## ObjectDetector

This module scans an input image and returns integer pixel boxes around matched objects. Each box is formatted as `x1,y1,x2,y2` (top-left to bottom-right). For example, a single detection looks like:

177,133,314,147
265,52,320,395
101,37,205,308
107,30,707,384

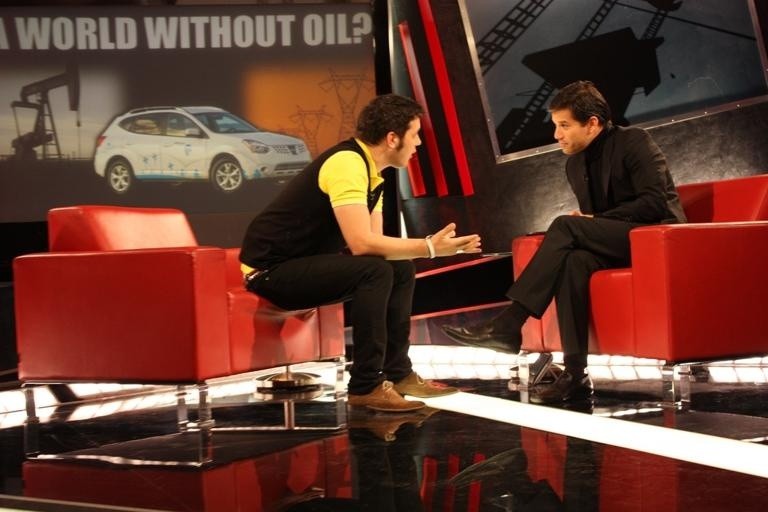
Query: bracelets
426,234,435,260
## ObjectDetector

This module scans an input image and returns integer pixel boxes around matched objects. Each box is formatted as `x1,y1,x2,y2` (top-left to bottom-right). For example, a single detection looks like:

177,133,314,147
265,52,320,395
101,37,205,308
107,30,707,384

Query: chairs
13,205,346,471
512,173,768,412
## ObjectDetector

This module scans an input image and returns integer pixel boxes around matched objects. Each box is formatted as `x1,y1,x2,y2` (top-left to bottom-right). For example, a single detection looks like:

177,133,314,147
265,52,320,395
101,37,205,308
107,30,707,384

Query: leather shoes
348,370,459,412
441,319,522,354
530,370,594,403
348,407,441,441
448,448,527,488
540,399,593,415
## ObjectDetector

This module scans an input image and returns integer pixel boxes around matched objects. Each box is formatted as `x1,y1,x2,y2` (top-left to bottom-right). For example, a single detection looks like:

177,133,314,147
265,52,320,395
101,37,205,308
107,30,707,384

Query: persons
343,403,440,510
240,93,481,412
440,82,688,406
448,404,597,511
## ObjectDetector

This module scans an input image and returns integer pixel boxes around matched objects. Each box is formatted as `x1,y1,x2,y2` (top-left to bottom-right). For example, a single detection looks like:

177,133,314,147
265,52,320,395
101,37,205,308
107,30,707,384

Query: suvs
91,103,314,192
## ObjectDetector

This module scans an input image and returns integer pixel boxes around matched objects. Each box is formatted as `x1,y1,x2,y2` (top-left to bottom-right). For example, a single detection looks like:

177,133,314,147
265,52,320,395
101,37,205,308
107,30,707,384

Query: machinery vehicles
11,65,80,159
